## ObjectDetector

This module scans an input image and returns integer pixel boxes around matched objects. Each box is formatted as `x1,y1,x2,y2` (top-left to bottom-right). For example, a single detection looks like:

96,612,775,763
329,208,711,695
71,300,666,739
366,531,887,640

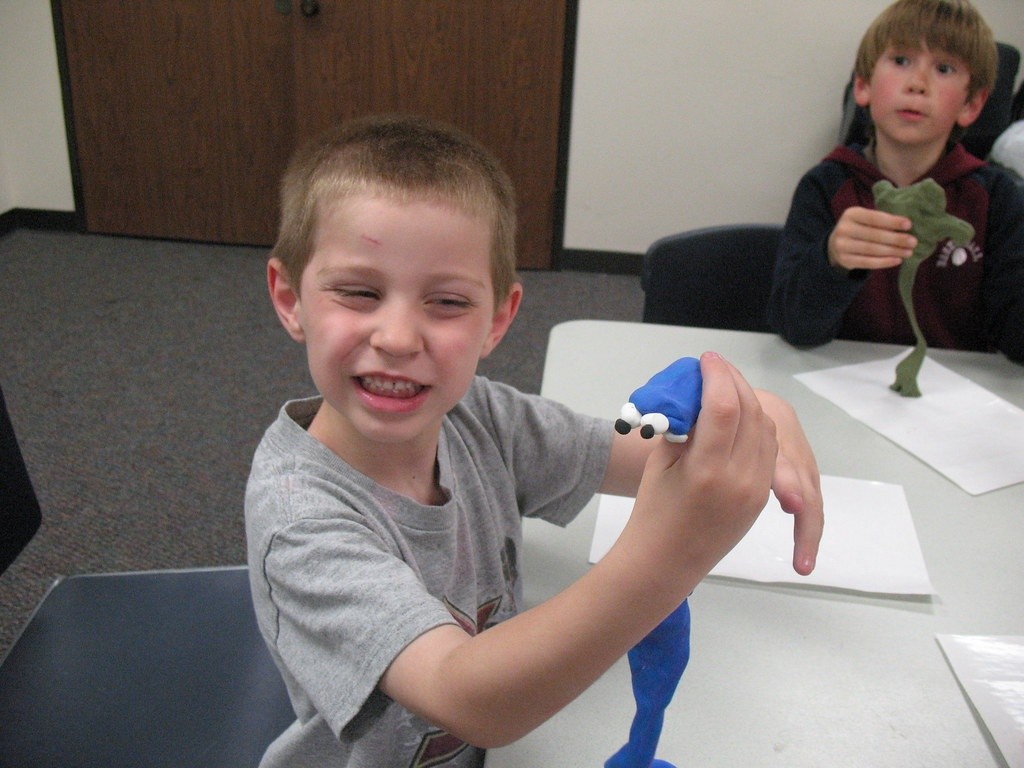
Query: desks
484,320,1024,767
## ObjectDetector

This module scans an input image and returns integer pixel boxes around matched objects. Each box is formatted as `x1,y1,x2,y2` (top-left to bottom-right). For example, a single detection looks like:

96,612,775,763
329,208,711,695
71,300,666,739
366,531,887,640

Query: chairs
1,382,300,768
642,221,786,330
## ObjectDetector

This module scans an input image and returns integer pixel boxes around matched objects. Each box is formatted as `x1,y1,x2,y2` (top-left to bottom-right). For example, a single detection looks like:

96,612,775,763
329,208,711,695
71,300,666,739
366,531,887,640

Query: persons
241,113,825,768
759,0,1024,365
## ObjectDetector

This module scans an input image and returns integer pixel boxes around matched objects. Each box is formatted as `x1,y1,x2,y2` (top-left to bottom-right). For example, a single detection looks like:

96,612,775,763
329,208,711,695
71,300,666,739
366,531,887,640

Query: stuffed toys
603,357,702,768
871,178,974,399
